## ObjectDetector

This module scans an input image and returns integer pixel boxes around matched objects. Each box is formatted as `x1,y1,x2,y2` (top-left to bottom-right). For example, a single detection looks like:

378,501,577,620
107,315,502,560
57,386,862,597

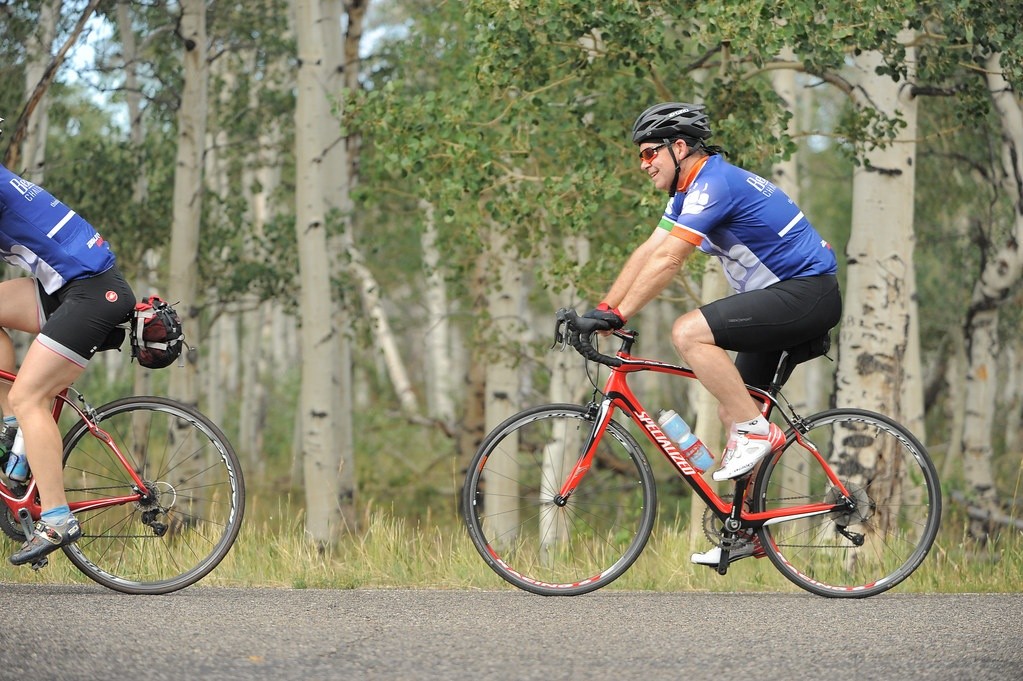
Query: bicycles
0,309,246,595
458,309,943,598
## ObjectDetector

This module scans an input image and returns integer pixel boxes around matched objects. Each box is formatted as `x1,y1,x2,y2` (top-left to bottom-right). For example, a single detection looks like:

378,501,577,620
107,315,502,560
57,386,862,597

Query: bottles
5,427,29,482
657,408,715,474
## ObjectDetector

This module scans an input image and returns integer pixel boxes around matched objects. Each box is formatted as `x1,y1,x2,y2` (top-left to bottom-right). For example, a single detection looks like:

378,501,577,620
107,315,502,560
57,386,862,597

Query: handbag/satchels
135,294,195,369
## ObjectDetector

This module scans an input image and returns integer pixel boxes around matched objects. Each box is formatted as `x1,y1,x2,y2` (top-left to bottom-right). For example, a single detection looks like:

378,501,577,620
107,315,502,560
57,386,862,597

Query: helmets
632,102,713,145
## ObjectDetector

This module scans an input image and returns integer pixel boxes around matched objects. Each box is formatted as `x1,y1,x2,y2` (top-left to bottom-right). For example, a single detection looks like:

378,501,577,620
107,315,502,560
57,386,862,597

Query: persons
581,101,843,571
0,162,137,565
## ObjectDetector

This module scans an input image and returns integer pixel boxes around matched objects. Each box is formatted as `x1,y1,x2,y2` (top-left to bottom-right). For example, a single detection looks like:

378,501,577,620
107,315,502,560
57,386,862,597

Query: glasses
639,142,673,164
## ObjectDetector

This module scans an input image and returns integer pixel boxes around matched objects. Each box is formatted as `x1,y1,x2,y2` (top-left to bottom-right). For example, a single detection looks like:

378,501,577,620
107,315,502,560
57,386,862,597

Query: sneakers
691,528,767,565
0,423,17,464
713,422,786,482
10,515,81,565
